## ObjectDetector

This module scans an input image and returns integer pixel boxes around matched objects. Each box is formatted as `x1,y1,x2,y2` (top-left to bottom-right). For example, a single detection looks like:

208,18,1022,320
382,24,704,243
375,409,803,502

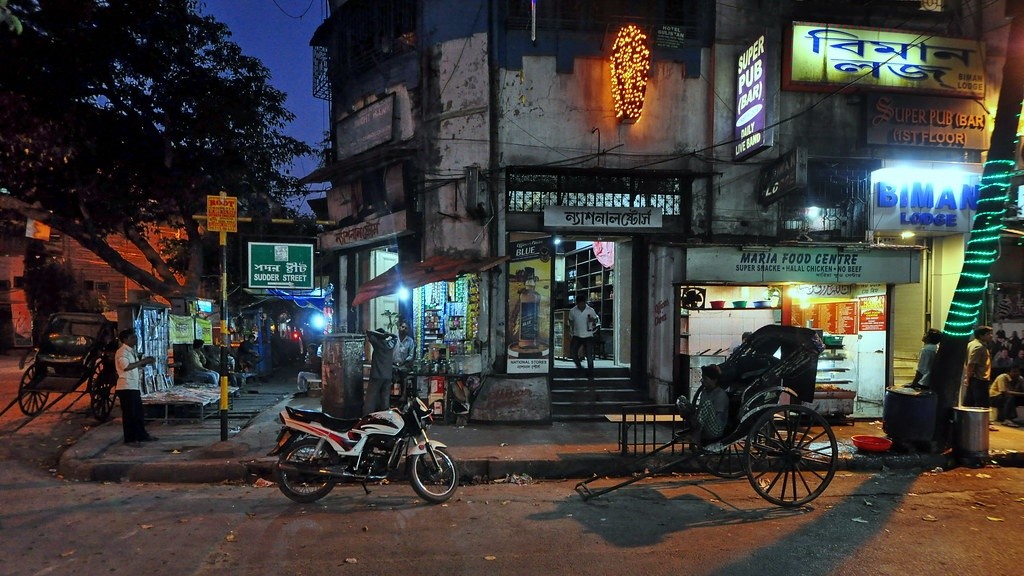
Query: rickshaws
0,312,118,434
573,323,839,508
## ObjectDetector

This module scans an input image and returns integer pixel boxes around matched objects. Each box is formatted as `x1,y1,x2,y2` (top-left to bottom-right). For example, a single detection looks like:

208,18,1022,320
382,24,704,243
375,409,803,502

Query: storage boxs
823,336,844,346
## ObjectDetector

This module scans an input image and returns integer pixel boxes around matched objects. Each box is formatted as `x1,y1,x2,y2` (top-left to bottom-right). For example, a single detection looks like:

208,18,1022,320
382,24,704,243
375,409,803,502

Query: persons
734,331,752,357
240,334,263,367
963,326,999,431
115,331,159,446
989,323,1024,428
180,340,219,386
905,329,940,388
674,365,730,444
393,322,415,402
569,296,602,385
364,329,398,412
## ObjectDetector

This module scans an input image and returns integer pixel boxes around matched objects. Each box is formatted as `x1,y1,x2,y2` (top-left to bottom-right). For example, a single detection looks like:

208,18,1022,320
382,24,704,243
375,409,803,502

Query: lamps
610,23,651,124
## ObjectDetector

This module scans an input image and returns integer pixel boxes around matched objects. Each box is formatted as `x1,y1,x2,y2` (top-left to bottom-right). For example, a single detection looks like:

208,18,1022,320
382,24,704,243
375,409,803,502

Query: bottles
519,268,541,348
417,352,464,374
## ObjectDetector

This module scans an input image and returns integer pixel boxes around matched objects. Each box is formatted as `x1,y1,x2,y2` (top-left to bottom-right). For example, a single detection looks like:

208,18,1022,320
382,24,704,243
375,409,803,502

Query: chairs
38,333,94,363
717,356,781,403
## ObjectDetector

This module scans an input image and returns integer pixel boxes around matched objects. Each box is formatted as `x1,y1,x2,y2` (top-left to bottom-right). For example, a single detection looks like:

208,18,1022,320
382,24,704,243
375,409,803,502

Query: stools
594,341,606,360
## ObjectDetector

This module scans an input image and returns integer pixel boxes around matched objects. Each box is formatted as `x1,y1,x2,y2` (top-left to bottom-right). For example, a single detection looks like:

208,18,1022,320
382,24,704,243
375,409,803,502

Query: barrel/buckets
952,407,992,452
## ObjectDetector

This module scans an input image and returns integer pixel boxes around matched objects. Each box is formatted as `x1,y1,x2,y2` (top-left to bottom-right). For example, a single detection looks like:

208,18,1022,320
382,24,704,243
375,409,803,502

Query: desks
605,414,685,450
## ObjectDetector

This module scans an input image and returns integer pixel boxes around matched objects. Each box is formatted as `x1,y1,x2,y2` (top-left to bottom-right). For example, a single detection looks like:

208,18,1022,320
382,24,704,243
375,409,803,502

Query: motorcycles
267,385,460,504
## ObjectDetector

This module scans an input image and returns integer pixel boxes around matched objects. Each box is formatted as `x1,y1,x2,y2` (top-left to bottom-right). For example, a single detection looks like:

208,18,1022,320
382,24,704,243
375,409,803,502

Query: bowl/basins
710,301,726,309
851,435,892,451
770,430,814,448
753,300,772,308
731,301,747,308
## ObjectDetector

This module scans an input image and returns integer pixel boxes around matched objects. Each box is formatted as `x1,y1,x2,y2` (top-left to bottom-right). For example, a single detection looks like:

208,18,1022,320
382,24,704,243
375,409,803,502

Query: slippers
676,431,700,444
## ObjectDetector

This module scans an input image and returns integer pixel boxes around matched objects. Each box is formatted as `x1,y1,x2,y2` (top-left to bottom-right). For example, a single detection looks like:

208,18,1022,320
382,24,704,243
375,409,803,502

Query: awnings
351,256,470,306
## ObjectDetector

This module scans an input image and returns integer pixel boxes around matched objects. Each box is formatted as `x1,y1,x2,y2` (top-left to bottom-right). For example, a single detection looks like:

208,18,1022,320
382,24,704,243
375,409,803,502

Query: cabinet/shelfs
565,244,613,354
800,346,856,415
554,311,570,360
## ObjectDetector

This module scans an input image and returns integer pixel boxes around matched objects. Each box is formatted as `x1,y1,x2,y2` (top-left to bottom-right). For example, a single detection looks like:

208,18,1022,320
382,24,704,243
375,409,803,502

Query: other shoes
142,436,159,441
1002,422,1019,427
989,426,999,431
124,441,140,447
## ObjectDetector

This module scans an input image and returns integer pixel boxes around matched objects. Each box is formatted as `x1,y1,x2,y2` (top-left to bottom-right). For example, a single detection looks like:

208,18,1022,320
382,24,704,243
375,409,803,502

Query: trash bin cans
951,407,992,467
